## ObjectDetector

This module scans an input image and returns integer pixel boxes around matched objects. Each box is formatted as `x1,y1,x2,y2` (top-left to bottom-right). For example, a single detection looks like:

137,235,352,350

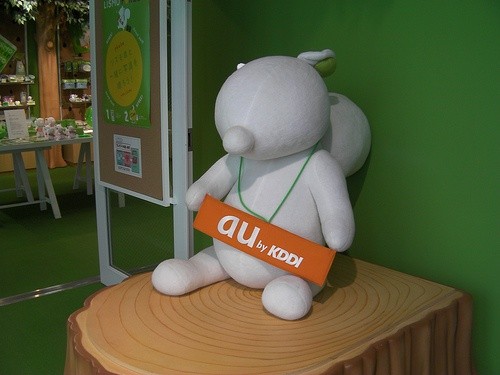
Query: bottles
16,62,25,82
20,92,27,104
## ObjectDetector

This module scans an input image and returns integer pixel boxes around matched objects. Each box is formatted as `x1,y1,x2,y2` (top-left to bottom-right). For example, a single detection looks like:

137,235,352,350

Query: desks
0,128,94,219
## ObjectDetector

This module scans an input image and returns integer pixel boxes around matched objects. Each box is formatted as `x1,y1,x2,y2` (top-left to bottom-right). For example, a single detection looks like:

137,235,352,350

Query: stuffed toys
148,48,373,322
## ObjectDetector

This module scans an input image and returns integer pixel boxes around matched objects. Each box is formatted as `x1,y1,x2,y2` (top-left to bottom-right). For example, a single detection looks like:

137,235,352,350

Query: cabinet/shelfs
0,80,35,121
